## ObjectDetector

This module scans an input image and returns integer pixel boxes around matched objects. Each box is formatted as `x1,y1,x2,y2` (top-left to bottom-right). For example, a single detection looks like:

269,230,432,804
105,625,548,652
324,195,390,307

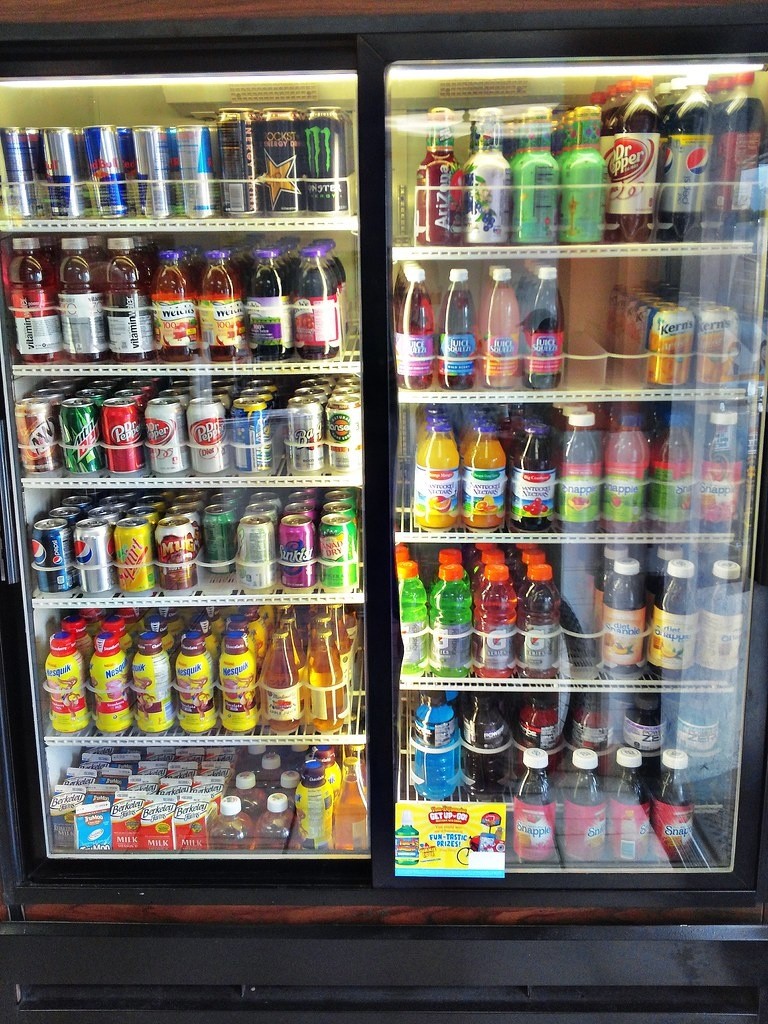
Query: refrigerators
0,6,768,1023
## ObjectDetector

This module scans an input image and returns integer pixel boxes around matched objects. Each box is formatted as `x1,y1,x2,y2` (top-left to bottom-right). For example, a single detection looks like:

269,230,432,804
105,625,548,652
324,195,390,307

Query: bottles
210,742,369,854
413,403,746,536
394,260,566,389
2,233,346,364
392,541,743,685
411,690,720,863
415,72,767,249
45,599,359,732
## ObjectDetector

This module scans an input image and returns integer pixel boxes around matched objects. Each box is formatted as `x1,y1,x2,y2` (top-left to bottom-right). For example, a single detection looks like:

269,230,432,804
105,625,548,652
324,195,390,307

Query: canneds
14,372,361,476
30,487,359,594
217,106,353,217
0,124,222,222
608,281,738,386
